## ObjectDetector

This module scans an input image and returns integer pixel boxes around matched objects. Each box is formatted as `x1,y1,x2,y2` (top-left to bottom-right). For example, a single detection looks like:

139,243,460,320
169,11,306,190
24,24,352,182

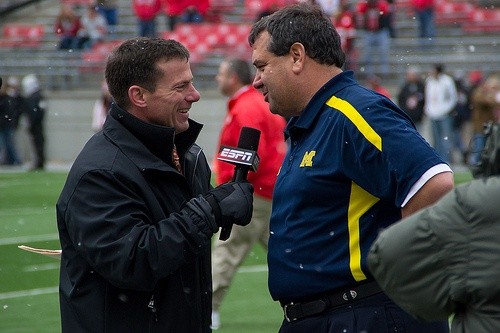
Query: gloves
205,178,255,227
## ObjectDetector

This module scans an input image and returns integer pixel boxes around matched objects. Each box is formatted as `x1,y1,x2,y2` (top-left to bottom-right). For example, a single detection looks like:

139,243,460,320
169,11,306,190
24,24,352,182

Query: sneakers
210,310,220,330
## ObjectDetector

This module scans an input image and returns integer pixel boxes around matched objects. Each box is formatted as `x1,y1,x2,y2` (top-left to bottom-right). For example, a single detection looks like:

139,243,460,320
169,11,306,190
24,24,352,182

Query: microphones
216,126,261,240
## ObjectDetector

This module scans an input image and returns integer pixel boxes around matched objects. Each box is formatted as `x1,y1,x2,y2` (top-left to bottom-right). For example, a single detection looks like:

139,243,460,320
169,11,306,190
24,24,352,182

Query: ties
171,144,184,174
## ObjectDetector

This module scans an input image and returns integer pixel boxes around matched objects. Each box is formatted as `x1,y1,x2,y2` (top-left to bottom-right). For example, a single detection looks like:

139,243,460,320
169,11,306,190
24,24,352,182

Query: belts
282,282,385,323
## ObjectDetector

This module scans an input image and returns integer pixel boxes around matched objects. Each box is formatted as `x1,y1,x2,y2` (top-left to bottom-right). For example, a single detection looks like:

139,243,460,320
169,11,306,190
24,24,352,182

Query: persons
398,65,426,138
92,81,114,132
334,1,438,79
451,68,500,164
22,74,47,172
212,59,287,330
366,176,500,333
55,0,234,51
245,1,340,22
370,74,391,99
0,77,23,166
56,37,255,333
248,5,454,333
423,63,458,164
